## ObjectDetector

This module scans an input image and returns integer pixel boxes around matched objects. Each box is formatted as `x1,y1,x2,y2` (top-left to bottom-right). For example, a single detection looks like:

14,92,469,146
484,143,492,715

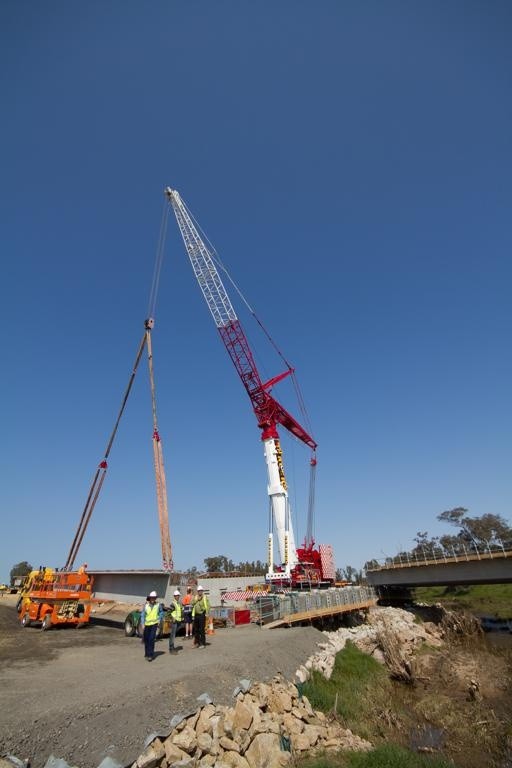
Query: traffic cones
207,616,214,635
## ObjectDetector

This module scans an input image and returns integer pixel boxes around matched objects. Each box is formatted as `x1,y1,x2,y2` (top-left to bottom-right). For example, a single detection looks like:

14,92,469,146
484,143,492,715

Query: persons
73,563,88,592
190,585,210,649
181,586,195,641
1,584,6,597
140,590,165,662
167,590,182,655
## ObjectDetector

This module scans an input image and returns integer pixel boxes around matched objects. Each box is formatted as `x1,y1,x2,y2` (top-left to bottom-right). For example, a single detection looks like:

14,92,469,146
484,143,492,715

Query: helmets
173,590,182,595
149,591,157,597
196,586,204,590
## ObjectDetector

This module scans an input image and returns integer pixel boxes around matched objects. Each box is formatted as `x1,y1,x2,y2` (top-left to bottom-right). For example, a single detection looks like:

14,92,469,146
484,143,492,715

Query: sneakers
192,643,205,649
182,636,193,641
145,655,153,661
170,649,179,655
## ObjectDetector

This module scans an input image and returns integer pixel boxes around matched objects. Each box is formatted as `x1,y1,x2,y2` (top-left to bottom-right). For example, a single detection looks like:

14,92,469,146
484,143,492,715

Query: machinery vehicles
65,186,335,592
16,567,94,632
